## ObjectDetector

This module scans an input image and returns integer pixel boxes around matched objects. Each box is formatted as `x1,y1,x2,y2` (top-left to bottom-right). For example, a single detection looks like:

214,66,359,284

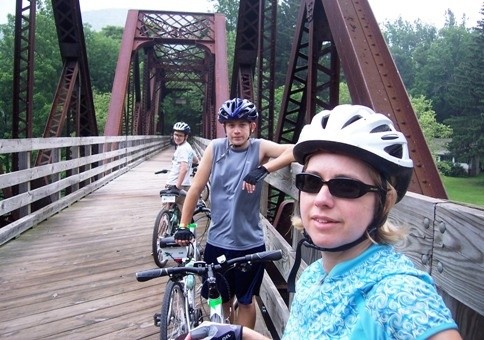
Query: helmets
291,104,414,204
217,97,258,125
172,122,190,135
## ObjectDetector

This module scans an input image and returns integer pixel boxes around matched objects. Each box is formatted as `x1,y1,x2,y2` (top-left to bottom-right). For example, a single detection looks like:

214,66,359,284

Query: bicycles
153,160,210,266
154,204,211,340
135,249,283,326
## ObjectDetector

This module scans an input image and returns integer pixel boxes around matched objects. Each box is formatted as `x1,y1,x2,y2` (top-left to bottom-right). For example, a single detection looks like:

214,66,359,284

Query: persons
164,121,199,214
184,104,463,340
173,96,298,331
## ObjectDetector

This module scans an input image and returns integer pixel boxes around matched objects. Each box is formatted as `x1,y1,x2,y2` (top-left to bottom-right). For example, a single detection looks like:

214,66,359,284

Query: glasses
294,173,383,200
